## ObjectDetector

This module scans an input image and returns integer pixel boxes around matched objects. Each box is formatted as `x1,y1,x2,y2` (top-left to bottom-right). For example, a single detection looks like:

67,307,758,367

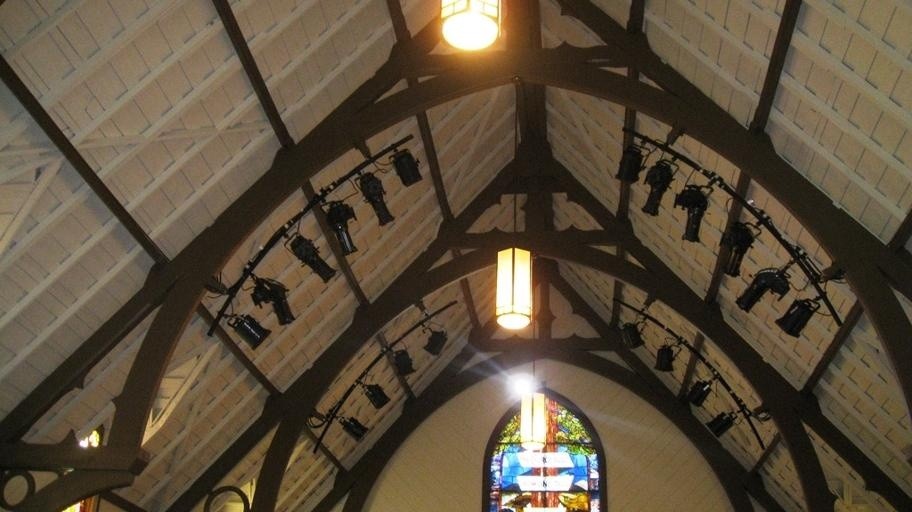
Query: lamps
440,1,501,51
519,392,547,449
496,248,533,329
623,316,646,347
333,416,369,440
228,311,271,351
640,159,678,214
722,222,763,276
771,297,820,341
423,325,450,358
291,235,337,285
359,380,390,409
327,200,358,257
733,260,794,311
390,147,423,187
705,411,741,438
675,179,713,241
652,342,682,372
393,348,417,377
362,170,394,225
684,378,715,406
611,141,650,182
250,278,295,326
